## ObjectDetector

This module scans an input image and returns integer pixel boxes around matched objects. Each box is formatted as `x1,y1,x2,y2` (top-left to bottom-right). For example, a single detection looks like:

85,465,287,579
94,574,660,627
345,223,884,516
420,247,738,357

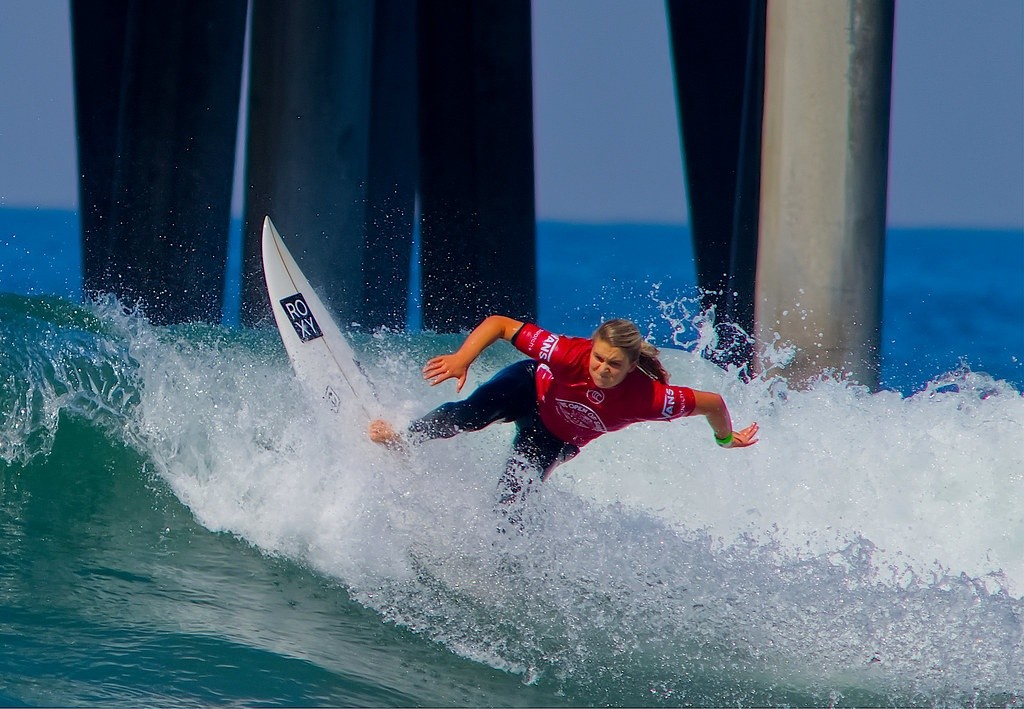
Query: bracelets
714,432,732,443
716,440,733,448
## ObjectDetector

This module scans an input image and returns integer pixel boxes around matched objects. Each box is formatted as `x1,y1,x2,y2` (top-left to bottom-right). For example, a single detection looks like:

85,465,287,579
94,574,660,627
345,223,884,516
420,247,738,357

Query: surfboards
262,216,390,422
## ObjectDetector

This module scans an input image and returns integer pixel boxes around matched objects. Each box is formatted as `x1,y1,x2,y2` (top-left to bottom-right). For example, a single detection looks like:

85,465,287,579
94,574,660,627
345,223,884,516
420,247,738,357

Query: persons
369,315,760,540
